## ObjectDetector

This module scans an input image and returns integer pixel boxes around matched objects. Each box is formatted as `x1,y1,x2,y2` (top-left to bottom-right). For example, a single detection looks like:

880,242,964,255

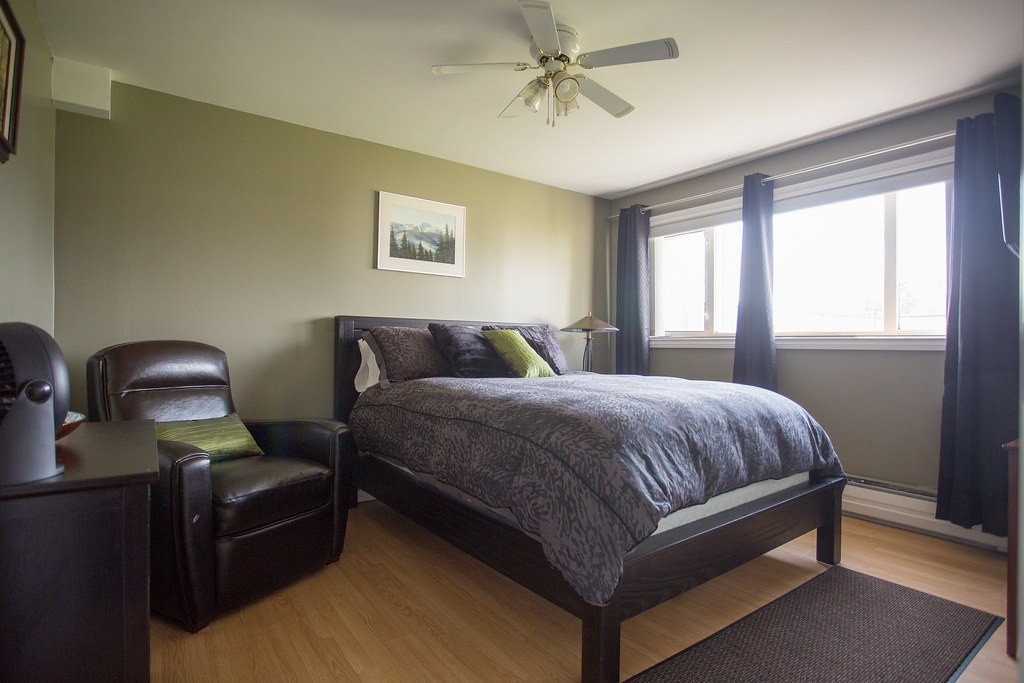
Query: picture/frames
0,0,28,165
376,189,467,278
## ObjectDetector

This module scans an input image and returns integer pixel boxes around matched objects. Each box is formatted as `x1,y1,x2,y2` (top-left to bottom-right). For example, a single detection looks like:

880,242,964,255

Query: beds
334,314,850,683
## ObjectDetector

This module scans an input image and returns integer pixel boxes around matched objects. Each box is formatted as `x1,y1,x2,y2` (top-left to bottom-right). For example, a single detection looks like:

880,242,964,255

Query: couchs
85,338,357,635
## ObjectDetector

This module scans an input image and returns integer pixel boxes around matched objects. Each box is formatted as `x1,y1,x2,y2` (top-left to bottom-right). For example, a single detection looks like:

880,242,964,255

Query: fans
429,0,681,121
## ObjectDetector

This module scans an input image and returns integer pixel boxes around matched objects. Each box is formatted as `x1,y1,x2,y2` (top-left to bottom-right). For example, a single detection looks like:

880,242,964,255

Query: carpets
619,562,1007,683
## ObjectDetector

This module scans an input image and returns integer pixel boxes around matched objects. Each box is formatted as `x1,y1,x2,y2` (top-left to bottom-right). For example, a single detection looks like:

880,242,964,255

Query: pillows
479,328,560,379
427,322,515,379
480,324,570,377
355,326,454,393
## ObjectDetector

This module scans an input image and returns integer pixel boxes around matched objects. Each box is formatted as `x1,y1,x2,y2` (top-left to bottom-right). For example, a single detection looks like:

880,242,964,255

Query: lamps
518,66,581,128
560,311,621,373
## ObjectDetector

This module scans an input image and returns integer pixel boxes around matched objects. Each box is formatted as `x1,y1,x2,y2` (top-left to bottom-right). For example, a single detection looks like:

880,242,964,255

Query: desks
1001,438,1022,657
0,419,159,683
